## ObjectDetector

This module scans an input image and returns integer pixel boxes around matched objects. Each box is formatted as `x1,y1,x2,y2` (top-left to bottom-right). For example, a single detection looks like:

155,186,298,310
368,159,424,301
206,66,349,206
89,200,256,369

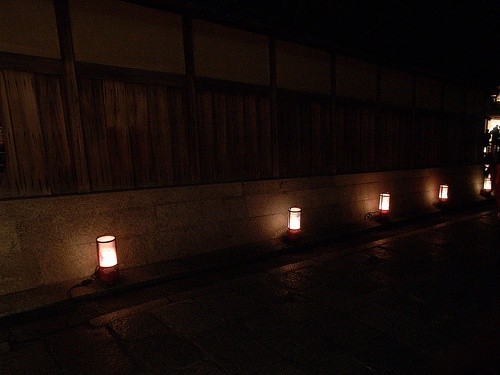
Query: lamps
284,207,302,247
374,192,391,223
95,235,121,286
438,185,449,209
482,177,492,197
